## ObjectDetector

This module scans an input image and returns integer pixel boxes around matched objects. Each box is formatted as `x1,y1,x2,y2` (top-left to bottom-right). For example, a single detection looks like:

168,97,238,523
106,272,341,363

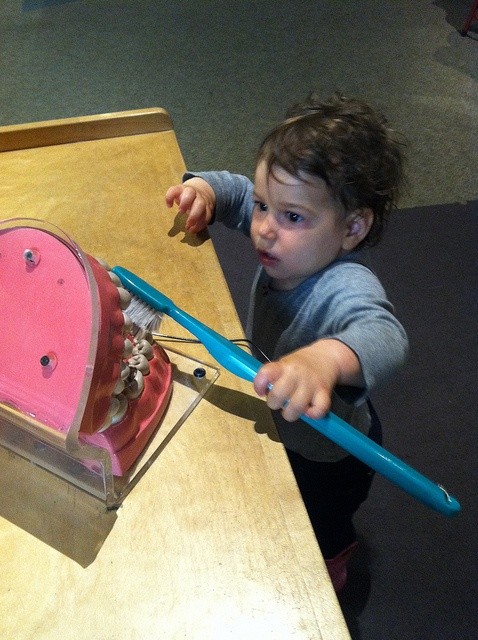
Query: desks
1,106,349,640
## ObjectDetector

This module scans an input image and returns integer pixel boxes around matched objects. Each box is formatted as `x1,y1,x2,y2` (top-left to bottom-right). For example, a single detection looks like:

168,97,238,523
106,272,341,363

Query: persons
166,90,411,592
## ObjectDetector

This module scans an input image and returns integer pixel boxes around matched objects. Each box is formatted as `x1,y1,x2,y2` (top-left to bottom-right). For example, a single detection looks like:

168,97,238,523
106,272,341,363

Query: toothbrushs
110,264,460,519
